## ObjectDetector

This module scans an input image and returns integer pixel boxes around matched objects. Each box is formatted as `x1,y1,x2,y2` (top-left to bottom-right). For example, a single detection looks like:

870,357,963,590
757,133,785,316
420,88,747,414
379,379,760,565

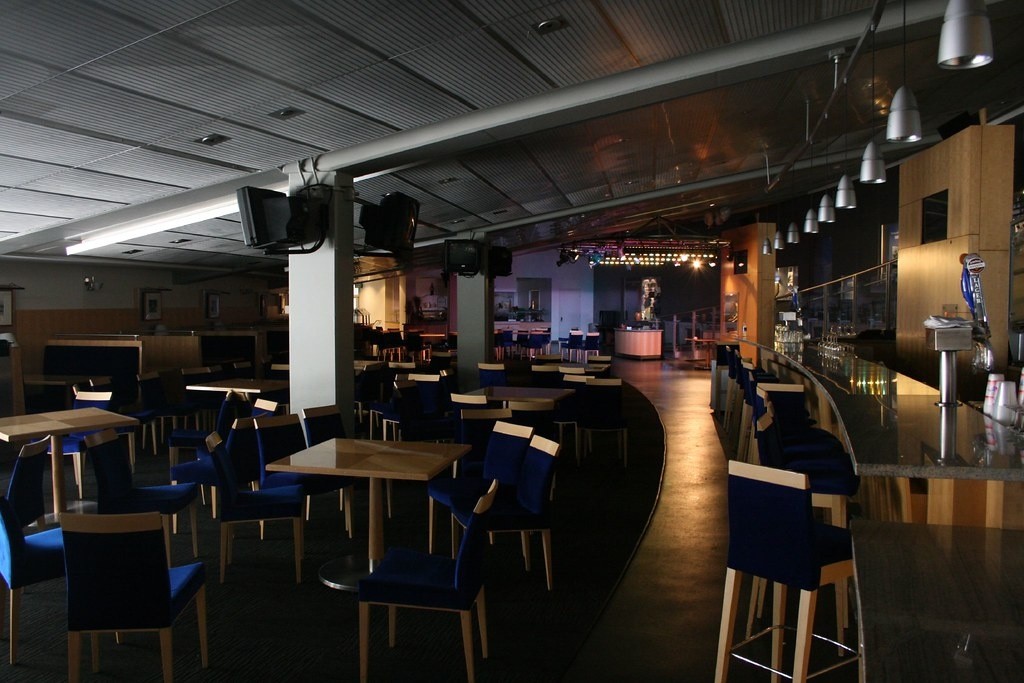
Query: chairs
714,342,863,683
0,323,623,683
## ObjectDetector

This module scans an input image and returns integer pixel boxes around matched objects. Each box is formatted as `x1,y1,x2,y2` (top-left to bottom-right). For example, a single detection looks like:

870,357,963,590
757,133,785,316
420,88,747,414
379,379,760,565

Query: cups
984,416,1024,463
983,368,1024,427
1014,412,1024,432
775,324,803,343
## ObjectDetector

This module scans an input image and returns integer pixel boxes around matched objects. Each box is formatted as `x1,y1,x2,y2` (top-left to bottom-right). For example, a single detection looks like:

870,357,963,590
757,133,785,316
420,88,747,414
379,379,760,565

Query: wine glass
818,333,840,359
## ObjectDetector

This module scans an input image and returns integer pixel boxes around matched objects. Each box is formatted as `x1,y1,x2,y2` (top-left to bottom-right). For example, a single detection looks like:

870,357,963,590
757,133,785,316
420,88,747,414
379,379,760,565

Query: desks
684,338,719,370
613,328,664,360
186,379,290,402
265,437,473,593
23,374,111,409
271,358,386,373
542,361,611,373
512,332,536,359
0,407,140,548
463,385,577,403
419,333,445,350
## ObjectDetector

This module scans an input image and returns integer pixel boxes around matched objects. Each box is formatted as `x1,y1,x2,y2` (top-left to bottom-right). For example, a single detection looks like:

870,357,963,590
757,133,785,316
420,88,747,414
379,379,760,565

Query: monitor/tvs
236,186,310,251
444,239,482,273
488,246,513,277
360,191,421,252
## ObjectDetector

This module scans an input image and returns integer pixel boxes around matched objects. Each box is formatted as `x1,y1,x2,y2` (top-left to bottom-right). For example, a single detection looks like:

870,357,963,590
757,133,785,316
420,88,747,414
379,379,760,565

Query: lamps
763,0,997,255
85,275,103,292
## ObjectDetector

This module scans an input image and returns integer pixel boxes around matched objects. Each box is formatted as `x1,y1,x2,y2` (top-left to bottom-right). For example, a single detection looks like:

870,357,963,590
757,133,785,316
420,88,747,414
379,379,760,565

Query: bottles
410,306,423,321
742,322,747,337
532,301,534,311
